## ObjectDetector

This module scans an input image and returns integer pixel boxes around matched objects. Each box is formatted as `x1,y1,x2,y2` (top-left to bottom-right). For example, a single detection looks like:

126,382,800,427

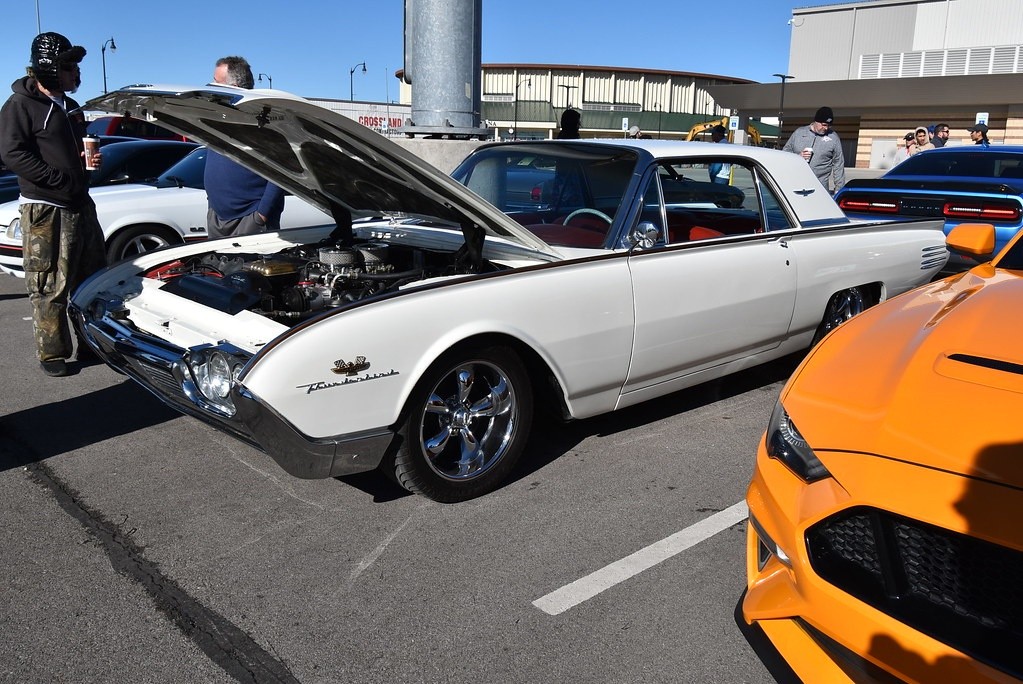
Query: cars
66,83,948,504
0,140,199,206
0,144,403,279
86,116,194,142
505,155,745,212
742,222,1023,684
832,141,1023,282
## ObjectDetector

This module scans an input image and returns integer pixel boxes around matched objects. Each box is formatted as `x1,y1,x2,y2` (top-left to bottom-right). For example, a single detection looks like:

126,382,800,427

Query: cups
803,148,813,163
82,134,101,171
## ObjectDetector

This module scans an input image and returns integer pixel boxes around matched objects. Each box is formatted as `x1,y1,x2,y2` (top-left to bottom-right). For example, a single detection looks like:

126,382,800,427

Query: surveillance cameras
788,22,791,25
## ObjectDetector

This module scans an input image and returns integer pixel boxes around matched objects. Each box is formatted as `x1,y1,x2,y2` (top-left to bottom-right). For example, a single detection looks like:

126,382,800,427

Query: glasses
942,130,949,134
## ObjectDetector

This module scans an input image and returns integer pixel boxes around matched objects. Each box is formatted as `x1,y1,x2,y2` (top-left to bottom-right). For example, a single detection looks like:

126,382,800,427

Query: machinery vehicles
684,118,765,147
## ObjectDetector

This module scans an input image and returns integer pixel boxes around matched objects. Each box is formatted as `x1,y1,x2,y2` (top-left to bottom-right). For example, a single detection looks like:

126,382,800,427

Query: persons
204,56,285,241
0,31,108,377
558,109,582,139
708,125,733,185
629,126,652,139
891,123,950,167
966,123,990,144
781,106,845,197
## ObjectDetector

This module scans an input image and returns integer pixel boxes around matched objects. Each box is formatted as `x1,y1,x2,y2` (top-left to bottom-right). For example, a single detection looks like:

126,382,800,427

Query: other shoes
41,359,67,376
75,349,102,361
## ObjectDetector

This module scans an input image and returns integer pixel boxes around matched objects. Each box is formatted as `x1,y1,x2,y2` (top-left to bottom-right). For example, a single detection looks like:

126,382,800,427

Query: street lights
654,102,662,139
351,62,367,102
558,85,579,109
773,74,796,150
258,73,272,89
102,36,116,94
514,79,531,140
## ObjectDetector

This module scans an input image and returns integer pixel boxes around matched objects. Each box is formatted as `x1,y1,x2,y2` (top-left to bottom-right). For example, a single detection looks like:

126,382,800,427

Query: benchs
595,205,762,243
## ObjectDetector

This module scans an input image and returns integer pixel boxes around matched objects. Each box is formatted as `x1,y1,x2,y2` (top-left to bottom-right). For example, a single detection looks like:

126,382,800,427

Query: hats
903,132,914,140
814,107,834,123
630,126,639,137
708,125,727,134
927,125,936,133
30,33,87,91
967,124,988,133
